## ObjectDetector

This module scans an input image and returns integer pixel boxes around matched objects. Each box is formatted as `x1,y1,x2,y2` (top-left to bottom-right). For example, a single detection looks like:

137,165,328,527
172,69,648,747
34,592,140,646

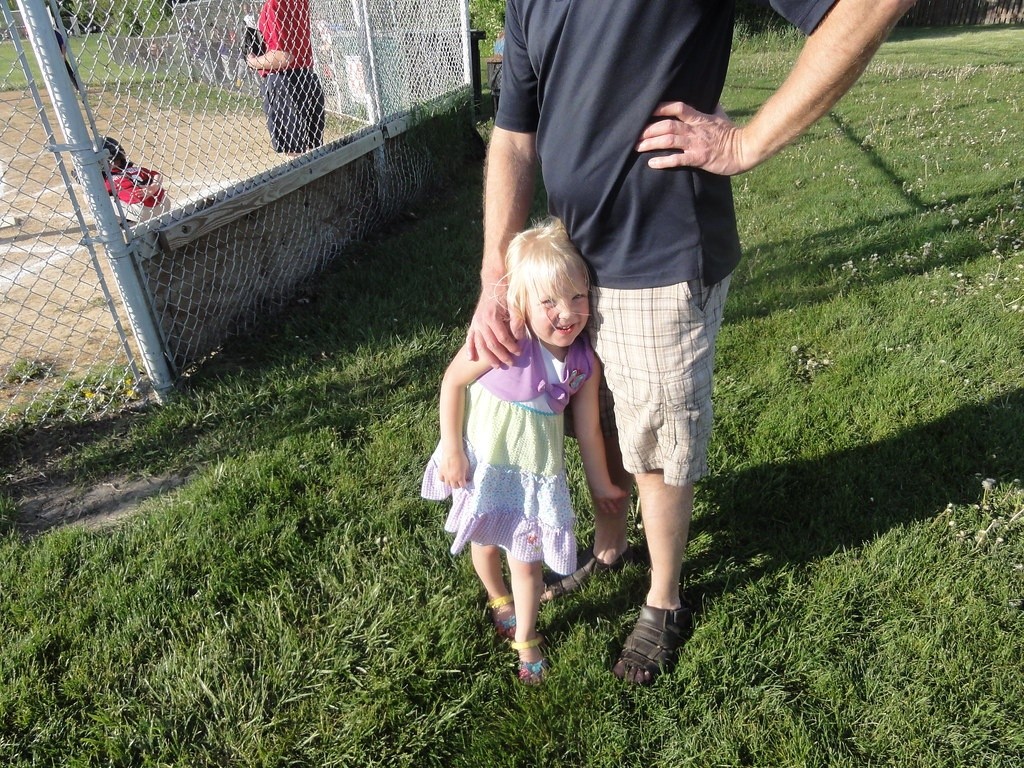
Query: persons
184,5,261,93
468,0,915,687
70,136,172,225
246,0,327,159
421,217,626,685
122,34,177,69
23,25,91,94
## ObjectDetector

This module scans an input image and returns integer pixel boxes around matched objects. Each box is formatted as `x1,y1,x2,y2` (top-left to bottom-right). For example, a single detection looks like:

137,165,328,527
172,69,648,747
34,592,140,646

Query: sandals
511,634,547,686
486,594,515,638
540,546,631,602
611,599,693,686
